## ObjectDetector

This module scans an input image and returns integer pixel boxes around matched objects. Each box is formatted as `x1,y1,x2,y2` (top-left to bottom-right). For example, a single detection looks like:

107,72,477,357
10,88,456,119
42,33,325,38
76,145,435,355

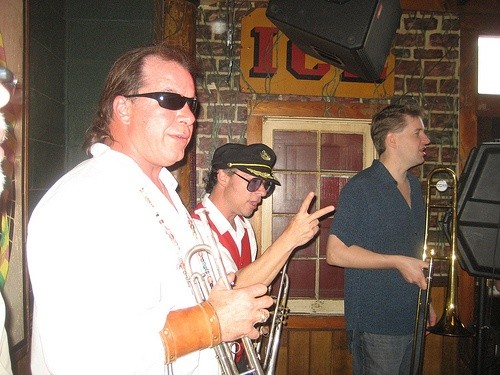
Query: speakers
443,141,500,279
265,0,404,82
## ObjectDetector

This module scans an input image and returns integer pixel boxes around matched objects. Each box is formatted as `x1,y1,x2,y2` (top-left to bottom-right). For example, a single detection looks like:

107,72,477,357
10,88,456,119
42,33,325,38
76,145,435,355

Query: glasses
119,92,199,116
228,170,271,193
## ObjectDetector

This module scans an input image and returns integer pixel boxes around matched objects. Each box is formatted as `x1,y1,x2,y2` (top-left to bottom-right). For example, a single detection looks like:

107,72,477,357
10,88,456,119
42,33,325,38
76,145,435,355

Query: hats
210,142,282,186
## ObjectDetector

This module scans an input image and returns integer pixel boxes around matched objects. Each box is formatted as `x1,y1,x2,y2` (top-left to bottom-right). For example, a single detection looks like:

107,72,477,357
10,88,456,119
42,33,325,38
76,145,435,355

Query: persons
189,144,335,375
326,105,432,375
25,45,275,375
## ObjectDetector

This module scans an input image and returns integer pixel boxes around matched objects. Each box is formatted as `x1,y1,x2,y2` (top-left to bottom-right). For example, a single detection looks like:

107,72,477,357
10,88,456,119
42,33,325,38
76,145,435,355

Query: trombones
409,168,470,375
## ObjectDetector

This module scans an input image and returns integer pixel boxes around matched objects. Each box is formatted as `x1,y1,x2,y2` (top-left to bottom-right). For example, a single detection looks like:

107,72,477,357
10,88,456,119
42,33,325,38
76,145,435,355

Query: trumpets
184,208,265,375
254,260,289,375
